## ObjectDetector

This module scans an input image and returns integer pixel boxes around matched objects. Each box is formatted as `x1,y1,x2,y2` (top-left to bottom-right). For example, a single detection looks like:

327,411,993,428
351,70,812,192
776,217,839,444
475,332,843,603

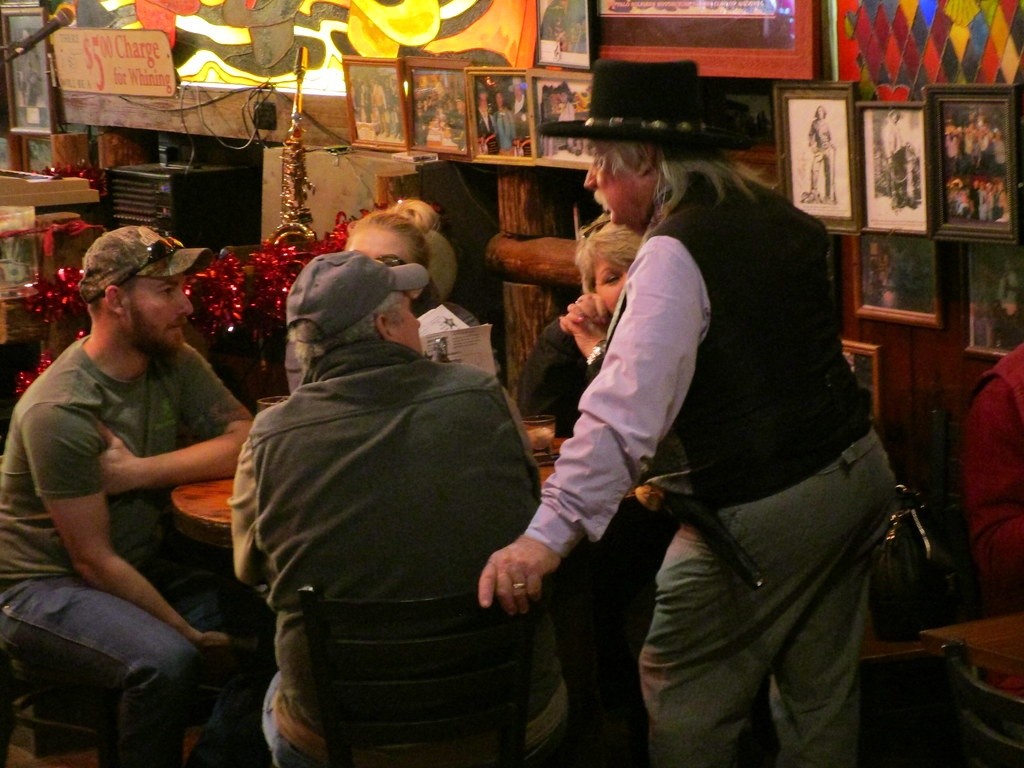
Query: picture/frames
21,135,51,174
774,82,865,235
537,0,595,70
464,65,536,167
850,228,946,331
841,339,887,447
856,98,929,237
1,7,57,136
594,0,822,82
404,56,475,166
922,80,1018,248
955,239,1024,359
525,69,598,171
342,54,410,153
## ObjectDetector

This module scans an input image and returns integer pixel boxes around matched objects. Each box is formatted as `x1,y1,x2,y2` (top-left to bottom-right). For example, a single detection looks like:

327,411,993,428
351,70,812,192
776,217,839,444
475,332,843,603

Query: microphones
4,5,78,62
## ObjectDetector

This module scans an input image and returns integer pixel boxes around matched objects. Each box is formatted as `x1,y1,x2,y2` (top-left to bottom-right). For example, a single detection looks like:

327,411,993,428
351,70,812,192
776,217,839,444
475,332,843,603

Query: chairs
296,583,541,768
0,649,220,768
858,410,1024,768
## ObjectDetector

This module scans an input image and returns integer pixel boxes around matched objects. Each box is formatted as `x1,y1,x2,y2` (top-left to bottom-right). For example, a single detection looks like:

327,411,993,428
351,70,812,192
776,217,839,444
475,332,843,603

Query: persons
516,215,684,491
945,111,1012,223
477,83,529,154
0,225,272,768
285,198,482,390
959,341,1024,700
540,87,595,158
350,75,403,138
232,251,566,767
478,60,902,768
417,75,466,150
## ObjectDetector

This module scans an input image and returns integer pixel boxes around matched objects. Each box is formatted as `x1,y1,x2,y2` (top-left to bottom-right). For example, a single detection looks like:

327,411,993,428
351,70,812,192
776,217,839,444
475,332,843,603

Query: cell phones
534,453,560,465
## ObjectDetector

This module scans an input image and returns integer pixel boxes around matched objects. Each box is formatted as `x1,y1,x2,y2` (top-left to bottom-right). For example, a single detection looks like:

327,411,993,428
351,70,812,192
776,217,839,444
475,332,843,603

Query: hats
535,58,753,152
285,249,429,344
77,225,213,303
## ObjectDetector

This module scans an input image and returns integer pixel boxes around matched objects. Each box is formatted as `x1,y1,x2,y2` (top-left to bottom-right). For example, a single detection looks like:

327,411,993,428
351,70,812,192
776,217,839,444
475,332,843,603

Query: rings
575,300,580,303
513,583,525,589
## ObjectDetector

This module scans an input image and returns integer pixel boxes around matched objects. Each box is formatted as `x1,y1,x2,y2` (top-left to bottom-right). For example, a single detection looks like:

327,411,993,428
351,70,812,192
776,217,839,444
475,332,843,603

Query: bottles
430,338,452,363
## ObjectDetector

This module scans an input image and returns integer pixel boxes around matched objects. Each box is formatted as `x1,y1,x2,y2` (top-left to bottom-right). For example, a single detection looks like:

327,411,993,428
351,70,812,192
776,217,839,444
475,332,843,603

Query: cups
523,416,555,450
257,396,291,413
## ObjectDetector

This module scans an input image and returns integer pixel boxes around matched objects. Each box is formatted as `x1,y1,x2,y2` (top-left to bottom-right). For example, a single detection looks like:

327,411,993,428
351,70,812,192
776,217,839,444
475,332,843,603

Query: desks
169,439,568,547
917,615,1024,679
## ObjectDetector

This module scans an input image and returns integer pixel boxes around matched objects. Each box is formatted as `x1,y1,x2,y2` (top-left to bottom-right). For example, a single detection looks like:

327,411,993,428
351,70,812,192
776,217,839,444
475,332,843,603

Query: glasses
375,255,408,267
117,235,185,287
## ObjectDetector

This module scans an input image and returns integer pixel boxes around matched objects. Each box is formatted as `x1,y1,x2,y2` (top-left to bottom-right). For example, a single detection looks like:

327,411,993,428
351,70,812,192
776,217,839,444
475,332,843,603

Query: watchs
586,339,606,365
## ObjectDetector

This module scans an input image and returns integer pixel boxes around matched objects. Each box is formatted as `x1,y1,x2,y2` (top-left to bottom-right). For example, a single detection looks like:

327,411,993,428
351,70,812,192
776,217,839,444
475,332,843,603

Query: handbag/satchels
869,483,947,644
